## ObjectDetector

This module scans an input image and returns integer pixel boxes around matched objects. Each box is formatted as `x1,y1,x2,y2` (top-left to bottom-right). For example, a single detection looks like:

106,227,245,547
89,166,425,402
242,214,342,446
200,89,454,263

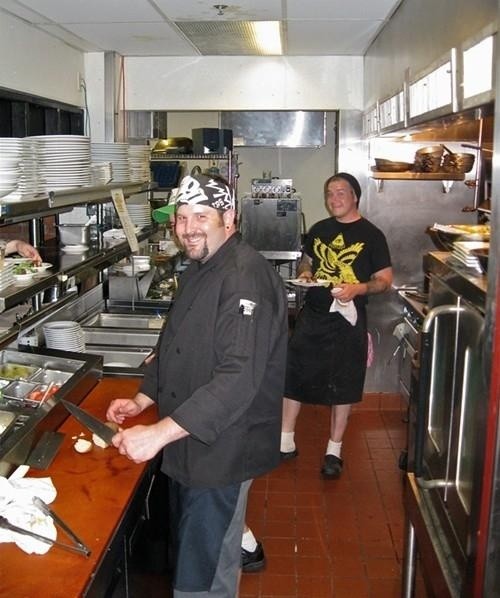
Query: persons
281,172,392,478
0,239,43,267
105,173,288,597
240,527,266,573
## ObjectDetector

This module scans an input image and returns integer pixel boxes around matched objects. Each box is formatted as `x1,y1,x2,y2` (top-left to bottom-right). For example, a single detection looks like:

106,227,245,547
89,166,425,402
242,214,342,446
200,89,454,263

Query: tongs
0,494,93,561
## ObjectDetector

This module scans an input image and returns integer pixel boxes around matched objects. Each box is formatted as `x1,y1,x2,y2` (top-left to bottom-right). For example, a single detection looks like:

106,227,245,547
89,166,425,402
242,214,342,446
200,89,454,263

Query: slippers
279,449,300,460
322,455,343,478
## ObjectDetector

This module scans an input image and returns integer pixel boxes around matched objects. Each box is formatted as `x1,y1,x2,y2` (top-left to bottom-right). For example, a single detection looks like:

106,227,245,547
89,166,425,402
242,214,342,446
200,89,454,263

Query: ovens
394,251,490,577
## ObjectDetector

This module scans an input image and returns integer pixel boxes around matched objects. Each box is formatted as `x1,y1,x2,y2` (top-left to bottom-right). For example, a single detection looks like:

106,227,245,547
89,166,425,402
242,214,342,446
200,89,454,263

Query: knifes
57,394,124,448
9,431,65,483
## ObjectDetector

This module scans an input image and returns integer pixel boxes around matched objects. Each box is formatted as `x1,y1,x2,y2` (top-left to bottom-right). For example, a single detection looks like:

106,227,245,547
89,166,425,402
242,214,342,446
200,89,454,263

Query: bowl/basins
13,272,33,284
25,261,54,275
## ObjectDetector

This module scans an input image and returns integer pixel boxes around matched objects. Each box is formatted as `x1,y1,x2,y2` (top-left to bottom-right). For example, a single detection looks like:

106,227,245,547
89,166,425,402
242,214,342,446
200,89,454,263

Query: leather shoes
241,540,267,571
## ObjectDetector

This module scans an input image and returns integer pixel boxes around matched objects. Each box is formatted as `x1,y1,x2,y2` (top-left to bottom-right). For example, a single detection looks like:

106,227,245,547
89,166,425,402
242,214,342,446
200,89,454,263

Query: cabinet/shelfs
0,177,178,359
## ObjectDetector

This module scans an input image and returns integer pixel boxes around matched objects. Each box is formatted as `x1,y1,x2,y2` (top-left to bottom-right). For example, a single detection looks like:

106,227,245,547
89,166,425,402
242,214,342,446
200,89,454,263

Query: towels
329,284,361,326
0,473,57,553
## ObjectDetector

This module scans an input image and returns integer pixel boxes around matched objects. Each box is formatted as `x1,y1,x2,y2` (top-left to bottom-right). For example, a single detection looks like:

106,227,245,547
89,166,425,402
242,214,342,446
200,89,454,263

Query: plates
9,256,32,265
285,277,331,290
448,238,488,270
375,156,412,171
127,144,152,185
1,257,16,292
123,201,153,228
61,244,90,255
88,141,131,187
0,160,21,199
42,320,87,355
1,134,38,197
29,131,92,195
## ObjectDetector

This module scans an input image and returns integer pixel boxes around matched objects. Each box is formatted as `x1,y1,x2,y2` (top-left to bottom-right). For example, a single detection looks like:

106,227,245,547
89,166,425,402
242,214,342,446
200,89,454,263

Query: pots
439,140,476,173
411,146,445,174
